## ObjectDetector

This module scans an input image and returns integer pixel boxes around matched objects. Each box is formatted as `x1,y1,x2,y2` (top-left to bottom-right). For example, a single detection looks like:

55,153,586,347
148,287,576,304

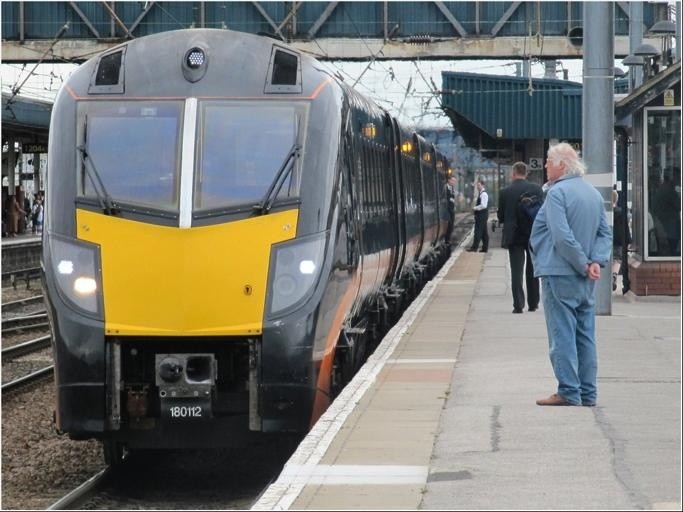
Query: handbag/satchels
517,191,542,237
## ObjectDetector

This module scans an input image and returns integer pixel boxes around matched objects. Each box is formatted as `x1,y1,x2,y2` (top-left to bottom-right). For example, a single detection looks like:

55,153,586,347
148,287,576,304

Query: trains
32,23,460,502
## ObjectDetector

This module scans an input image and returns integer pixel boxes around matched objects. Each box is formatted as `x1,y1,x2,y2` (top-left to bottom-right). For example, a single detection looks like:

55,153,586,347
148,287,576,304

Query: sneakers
613,272,618,291
467,248,487,252
537,393,568,406
512,305,539,313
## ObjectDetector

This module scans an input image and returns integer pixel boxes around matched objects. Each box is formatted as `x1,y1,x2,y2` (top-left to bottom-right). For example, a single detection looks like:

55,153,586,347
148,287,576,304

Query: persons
464,180,489,253
524,140,614,408
1,188,43,239
444,175,457,242
610,188,630,293
496,160,545,313
651,165,681,256
